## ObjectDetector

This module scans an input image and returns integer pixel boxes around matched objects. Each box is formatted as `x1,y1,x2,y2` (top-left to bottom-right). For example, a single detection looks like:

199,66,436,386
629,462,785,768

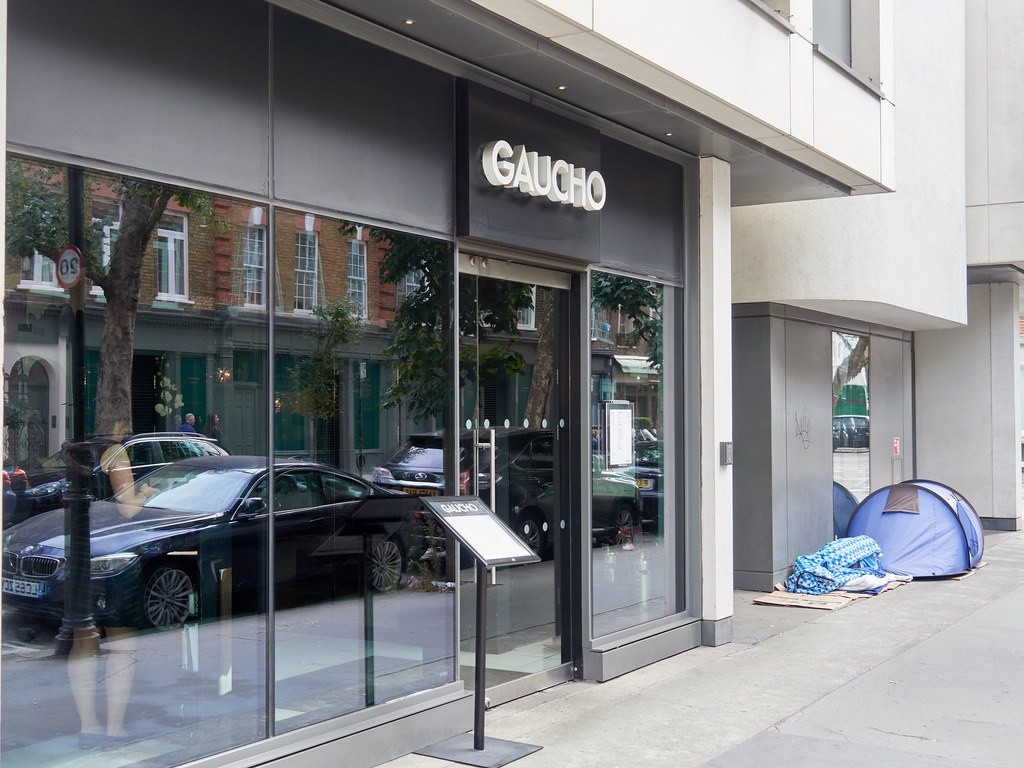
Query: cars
2,454,437,633
634,439,663,532
832,414,870,451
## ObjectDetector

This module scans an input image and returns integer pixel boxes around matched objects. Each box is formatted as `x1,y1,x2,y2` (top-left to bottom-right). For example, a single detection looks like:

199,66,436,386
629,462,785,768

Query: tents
833,479,860,540
845,479,985,579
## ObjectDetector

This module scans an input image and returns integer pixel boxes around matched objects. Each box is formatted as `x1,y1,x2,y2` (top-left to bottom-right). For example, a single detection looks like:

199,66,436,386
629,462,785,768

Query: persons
205,413,223,444
169,412,197,438
61,410,161,753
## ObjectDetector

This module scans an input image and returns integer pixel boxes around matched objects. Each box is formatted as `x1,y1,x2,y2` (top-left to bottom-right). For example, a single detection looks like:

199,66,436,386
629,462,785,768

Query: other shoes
79,726,143,750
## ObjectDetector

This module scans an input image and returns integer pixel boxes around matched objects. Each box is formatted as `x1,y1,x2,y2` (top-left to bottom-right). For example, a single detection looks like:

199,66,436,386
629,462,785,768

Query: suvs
8,430,231,528
370,423,645,563
